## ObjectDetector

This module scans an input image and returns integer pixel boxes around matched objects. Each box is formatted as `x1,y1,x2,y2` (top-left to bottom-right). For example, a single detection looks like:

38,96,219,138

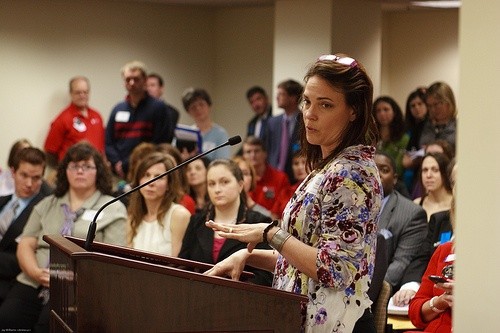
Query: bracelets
268,229,292,253
263,219,278,244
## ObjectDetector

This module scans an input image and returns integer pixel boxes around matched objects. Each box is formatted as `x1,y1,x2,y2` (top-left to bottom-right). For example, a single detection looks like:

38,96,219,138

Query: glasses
68,162,98,172
319,54,358,69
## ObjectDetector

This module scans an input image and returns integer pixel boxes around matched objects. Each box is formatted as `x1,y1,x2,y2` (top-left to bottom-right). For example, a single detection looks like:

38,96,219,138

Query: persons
121,152,194,258
2,141,130,332
0,147,56,307
178,157,276,286
371,82,455,332
0,59,313,223
205,52,388,333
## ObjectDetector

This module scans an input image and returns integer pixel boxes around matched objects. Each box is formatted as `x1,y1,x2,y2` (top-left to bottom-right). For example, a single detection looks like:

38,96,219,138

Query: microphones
85,136,242,251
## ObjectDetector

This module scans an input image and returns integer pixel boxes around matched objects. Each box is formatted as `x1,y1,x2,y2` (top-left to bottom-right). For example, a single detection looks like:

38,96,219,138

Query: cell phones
428,274,447,284
